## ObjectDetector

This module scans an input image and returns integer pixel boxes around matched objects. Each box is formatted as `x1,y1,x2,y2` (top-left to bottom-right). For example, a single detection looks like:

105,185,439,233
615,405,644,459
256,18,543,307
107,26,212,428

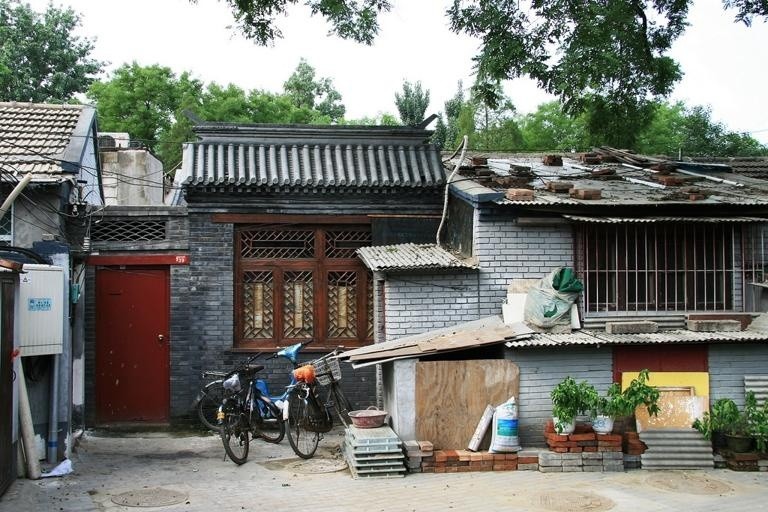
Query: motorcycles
196,339,313,430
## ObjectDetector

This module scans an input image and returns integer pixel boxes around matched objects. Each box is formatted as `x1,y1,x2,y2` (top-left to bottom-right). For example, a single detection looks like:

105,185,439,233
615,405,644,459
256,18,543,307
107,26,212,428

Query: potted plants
692,387,768,452
548,367,662,436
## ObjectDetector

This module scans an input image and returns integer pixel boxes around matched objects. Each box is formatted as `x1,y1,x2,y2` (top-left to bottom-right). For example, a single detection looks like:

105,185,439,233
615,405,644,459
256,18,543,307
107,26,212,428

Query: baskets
312,357,342,386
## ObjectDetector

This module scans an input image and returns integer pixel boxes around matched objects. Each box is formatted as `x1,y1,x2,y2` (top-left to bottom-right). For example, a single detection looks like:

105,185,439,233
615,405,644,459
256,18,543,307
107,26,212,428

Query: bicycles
215,352,284,464
283,345,354,460
